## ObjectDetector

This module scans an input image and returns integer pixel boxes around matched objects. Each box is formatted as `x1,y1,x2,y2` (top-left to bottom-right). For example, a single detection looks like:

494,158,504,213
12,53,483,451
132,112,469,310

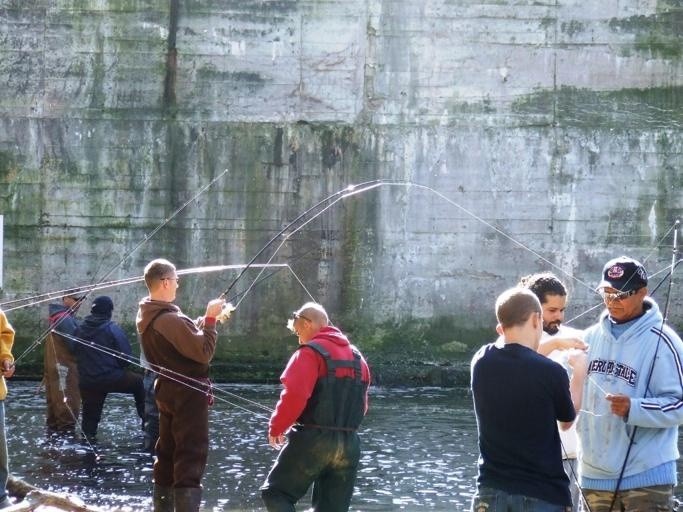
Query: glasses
601,289,637,299
294,312,311,322
160,277,179,283
69,295,85,302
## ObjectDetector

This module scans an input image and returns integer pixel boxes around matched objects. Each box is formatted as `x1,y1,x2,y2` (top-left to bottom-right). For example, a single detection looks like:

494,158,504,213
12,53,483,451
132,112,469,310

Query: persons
495,270,585,512
42,286,86,441
561,257,682,511
257,303,370,512
70,296,146,449
139,302,235,457
0,308,16,507
134,258,227,512
470,287,590,511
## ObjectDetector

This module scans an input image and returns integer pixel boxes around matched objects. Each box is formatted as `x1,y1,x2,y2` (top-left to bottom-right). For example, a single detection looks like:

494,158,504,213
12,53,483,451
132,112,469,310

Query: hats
595,256,648,291
92,295,112,315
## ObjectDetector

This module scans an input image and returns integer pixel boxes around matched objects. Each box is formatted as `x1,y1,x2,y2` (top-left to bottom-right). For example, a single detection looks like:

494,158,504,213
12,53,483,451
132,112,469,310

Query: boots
174,487,202,511
153,484,174,511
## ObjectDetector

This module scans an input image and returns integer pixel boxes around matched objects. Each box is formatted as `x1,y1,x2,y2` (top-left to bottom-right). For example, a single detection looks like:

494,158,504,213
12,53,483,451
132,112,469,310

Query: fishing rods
1,168,230,381
216,178,437,309
1,261,290,311
561,221,683,507
48,326,304,430
